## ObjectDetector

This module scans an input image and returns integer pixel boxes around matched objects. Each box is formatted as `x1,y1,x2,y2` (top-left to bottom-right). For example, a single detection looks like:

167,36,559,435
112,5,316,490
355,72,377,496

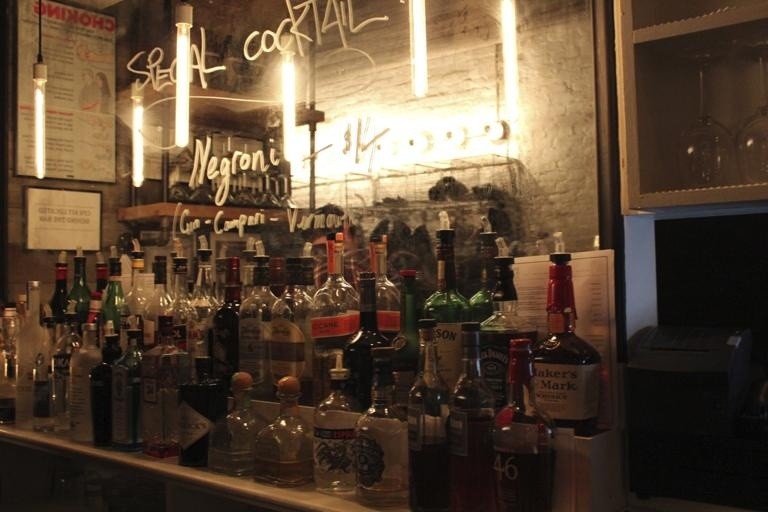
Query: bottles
1,210,602,512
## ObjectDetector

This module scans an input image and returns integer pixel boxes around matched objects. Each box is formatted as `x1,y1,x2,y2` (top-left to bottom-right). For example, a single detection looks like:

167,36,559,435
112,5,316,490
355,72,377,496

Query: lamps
130,1,144,187
32,0,48,181
171,0,192,147
407,0,430,96
276,30,300,164
497,0,523,113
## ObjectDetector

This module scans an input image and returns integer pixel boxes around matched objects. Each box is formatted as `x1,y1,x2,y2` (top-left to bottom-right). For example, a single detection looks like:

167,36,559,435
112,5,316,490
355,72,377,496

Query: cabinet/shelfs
609,1,767,213
115,83,323,221
288,149,525,249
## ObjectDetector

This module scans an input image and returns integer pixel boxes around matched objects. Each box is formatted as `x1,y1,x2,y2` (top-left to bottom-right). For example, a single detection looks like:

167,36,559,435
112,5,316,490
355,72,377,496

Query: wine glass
662,35,768,192
